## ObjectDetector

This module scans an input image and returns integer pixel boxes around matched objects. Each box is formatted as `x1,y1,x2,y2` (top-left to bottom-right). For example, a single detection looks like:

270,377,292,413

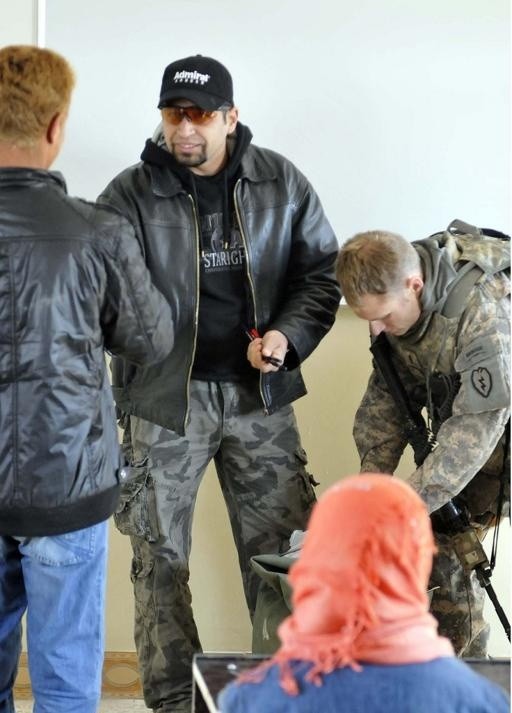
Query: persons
328,214,510,666
0,40,178,713
215,467,510,713
88,51,342,712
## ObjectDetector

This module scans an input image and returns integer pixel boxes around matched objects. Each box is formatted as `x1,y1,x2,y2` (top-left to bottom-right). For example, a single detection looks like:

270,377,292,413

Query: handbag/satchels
250,547,298,654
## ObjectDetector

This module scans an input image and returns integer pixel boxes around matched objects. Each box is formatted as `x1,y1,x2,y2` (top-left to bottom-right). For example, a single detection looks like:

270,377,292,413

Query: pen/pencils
245,328,287,371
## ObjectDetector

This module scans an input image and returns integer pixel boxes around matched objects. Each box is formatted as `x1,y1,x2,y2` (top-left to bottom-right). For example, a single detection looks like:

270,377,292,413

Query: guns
368,329,510,642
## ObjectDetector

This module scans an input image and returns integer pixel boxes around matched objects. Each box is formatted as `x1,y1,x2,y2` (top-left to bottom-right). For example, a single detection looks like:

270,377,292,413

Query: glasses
161,106,218,125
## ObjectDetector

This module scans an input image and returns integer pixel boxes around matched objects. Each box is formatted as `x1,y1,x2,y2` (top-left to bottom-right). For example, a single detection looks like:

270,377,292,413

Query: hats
157,54,233,111
